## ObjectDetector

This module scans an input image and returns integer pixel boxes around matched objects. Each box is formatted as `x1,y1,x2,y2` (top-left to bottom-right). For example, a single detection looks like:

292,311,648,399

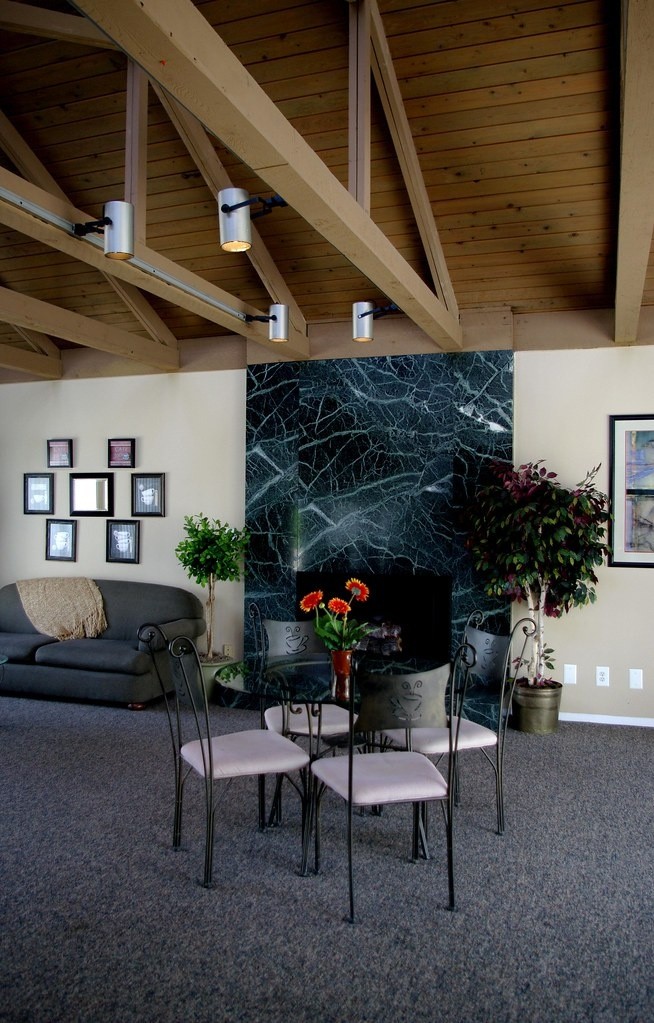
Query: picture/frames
47,439,73,468
46,519,77,562
106,519,140,564
23,472,54,515
131,473,165,517
608,414,654,568
108,438,136,468
69,473,114,517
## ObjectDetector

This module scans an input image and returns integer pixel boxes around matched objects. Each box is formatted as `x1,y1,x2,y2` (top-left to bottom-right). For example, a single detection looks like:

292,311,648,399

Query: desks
216,653,478,875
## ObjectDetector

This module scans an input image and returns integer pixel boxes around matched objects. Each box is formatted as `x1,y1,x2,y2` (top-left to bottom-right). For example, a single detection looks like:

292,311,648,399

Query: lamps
217,188,289,252
245,302,289,343
352,302,402,343
73,200,134,260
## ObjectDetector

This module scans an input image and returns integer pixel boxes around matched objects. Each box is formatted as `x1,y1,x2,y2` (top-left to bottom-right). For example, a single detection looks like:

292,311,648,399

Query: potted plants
466,459,615,736
173,513,251,706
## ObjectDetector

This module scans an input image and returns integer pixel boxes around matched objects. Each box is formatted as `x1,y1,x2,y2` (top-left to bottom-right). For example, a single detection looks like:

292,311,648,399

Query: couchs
0,577,206,711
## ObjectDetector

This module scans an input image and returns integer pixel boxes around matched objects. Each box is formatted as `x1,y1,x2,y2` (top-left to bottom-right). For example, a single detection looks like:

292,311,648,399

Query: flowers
299,578,380,650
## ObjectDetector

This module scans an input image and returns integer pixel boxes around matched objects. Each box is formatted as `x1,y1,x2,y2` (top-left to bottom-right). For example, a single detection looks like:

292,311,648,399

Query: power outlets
596,666,609,686
223,644,234,657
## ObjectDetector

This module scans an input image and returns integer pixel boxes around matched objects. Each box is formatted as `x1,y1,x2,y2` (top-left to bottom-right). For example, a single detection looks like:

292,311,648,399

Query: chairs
248,603,367,825
137,622,310,889
311,644,478,925
379,610,537,841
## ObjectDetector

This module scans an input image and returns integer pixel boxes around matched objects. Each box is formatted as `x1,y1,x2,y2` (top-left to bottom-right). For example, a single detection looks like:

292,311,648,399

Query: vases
332,650,352,699
